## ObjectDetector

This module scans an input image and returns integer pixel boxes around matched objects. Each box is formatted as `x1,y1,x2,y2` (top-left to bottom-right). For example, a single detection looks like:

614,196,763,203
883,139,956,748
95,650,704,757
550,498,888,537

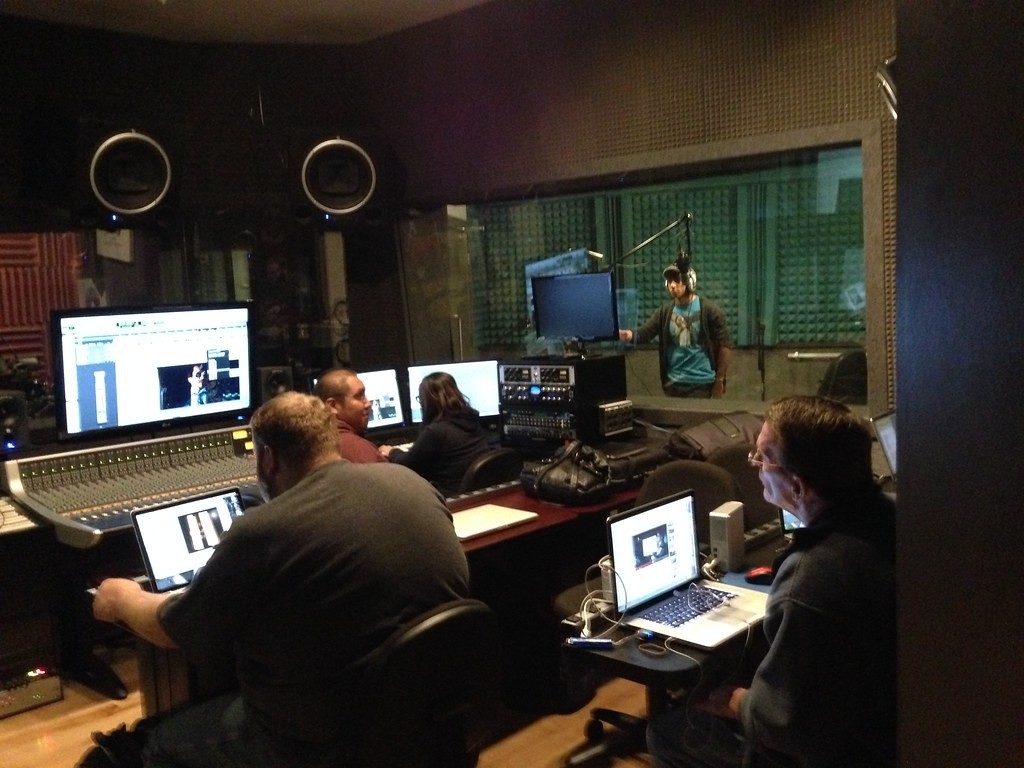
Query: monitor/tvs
405,360,502,425
49,300,260,445
313,368,405,431
531,271,619,343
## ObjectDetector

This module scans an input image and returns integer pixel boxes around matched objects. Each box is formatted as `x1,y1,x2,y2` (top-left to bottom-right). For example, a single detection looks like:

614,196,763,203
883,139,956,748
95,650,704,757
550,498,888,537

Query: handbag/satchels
520,437,611,507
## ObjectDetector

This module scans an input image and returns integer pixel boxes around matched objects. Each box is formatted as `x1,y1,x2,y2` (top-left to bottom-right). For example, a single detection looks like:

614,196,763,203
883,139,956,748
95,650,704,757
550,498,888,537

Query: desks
0,392,897,768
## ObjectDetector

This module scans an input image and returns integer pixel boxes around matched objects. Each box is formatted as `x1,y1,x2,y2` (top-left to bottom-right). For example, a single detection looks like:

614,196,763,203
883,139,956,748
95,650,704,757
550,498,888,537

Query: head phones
665,269,697,292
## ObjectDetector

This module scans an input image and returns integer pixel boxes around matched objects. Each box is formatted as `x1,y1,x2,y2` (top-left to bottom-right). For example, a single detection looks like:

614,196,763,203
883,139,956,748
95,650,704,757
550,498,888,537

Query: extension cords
561,601,613,630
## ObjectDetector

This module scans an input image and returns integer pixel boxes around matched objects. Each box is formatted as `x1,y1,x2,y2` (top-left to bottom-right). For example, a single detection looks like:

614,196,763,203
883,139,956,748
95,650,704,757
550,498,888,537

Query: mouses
744,567,772,586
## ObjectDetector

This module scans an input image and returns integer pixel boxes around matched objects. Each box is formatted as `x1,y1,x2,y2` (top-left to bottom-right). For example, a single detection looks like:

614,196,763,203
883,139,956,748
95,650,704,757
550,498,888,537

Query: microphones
679,250,690,285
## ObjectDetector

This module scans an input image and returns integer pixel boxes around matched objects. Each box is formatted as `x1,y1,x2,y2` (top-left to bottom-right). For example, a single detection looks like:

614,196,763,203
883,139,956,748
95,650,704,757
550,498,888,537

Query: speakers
709,500,746,573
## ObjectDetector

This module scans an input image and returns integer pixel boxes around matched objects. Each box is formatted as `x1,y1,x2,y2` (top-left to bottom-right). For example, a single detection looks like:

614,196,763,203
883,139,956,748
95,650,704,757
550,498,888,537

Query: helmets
662,261,697,292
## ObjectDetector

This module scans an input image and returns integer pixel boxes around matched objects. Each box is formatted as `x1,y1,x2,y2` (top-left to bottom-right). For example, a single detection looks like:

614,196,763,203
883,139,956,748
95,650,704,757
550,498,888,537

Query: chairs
704,444,781,531
633,460,745,543
336,600,499,768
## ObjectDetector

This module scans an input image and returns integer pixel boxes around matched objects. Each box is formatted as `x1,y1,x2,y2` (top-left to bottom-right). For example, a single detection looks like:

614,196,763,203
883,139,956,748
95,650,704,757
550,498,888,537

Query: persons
312,366,389,463
618,261,733,399
93,388,479,768
168,364,223,408
378,373,491,497
648,394,896,768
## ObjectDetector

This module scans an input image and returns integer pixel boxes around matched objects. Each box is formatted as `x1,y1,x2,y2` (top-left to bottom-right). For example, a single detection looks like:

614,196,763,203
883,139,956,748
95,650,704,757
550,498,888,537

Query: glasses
747,449,787,471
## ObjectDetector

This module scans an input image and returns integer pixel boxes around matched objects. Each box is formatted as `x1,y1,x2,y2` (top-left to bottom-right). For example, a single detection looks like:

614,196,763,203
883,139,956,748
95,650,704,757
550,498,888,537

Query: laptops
779,508,806,541
608,488,768,652
871,407,898,482
451,503,539,542
130,486,246,594
